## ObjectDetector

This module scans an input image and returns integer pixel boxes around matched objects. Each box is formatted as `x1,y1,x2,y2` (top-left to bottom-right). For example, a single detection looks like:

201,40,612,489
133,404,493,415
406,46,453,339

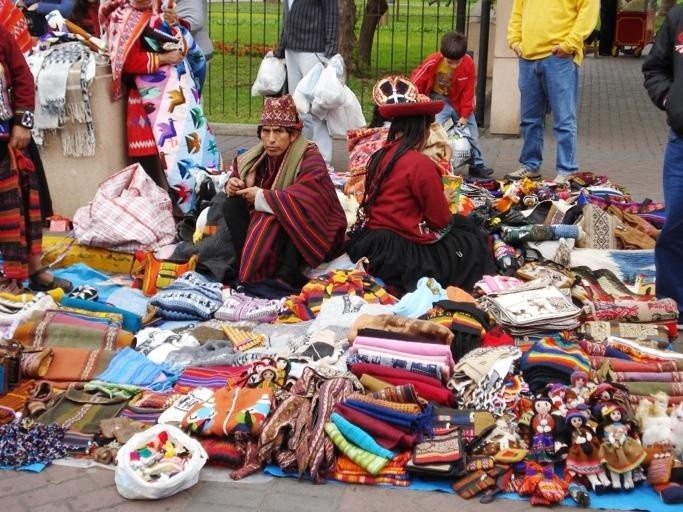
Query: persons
642,0,682,332
218,92,350,295
96,0,225,219
341,73,491,299
0,21,71,293
274,1,341,152
504,0,600,187
409,31,494,182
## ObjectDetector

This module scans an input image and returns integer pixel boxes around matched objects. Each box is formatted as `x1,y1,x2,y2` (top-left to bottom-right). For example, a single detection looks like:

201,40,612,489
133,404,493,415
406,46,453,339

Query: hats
372,75,419,106
378,95,445,121
256,94,304,128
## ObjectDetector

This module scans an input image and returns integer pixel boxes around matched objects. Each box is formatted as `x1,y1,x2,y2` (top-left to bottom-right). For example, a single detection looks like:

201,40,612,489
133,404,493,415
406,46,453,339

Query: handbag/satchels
38,162,177,269
445,123,474,170
130,249,198,297
18,5,48,36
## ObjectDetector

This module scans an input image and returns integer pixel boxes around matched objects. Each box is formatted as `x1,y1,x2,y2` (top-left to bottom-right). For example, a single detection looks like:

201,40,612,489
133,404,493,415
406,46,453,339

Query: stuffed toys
236,357,291,393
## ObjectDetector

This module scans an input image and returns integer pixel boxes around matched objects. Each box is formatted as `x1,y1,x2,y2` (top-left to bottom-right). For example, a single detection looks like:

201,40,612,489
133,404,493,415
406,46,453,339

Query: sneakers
504,167,540,181
554,172,572,183
29,278,73,293
469,165,493,176
